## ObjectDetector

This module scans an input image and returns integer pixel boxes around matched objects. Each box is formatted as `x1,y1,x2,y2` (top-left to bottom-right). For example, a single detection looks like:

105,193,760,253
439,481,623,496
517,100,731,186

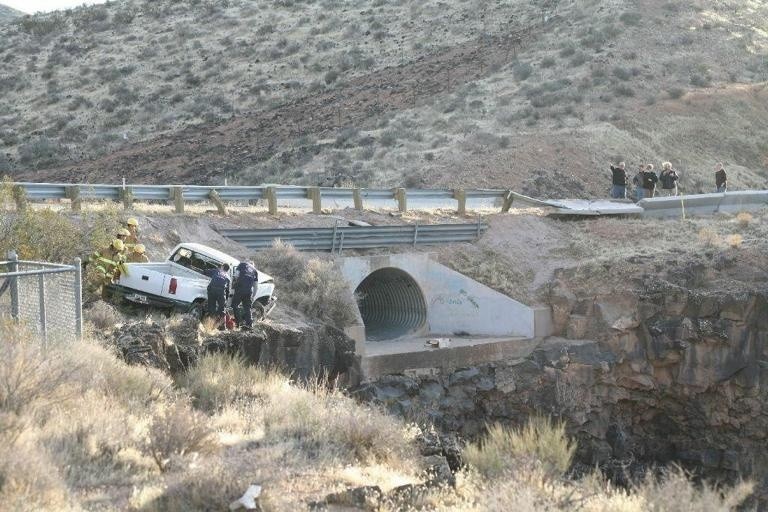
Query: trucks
102,240,277,325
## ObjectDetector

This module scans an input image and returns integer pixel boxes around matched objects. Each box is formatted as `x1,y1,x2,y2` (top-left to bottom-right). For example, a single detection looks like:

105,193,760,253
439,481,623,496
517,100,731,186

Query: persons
659,162,680,197
92,215,150,279
202,263,232,319
643,164,658,198
714,161,728,193
609,161,629,200
633,164,647,202
230,259,260,330
